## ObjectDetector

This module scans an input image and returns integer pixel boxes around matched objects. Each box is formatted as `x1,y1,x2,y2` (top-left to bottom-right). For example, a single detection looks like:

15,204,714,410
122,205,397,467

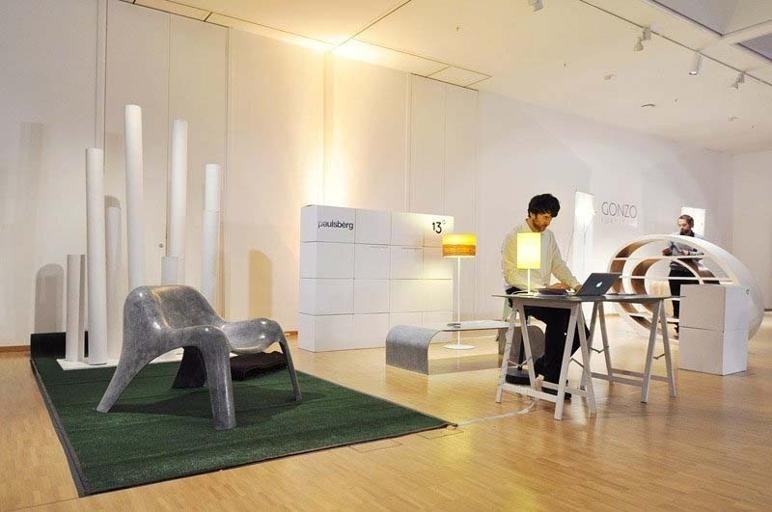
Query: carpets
31,331,453,495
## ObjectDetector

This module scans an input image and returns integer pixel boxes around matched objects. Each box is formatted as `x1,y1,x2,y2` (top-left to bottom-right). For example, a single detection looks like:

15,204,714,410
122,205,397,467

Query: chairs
93,285,305,427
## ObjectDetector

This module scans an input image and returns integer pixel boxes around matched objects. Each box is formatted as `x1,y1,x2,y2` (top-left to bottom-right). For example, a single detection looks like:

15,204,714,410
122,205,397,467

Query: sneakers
534,358,572,400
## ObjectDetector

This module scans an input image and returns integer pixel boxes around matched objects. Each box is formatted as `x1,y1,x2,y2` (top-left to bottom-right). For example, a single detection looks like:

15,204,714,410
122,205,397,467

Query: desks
493,292,684,414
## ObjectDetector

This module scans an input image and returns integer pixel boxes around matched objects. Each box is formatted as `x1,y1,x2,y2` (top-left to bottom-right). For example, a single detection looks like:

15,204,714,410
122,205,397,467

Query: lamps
633,27,751,90
515,231,542,294
442,235,477,323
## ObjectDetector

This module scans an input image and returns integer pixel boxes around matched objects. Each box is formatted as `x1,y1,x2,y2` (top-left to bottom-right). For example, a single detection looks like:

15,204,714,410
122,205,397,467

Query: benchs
386,319,546,375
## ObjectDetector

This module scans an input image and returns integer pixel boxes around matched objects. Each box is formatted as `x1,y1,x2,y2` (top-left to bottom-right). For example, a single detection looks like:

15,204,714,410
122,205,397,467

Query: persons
500,192,589,398
663,215,705,336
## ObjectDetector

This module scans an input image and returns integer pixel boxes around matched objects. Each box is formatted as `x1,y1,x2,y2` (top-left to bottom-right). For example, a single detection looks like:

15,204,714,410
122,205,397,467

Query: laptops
569,272,622,296
